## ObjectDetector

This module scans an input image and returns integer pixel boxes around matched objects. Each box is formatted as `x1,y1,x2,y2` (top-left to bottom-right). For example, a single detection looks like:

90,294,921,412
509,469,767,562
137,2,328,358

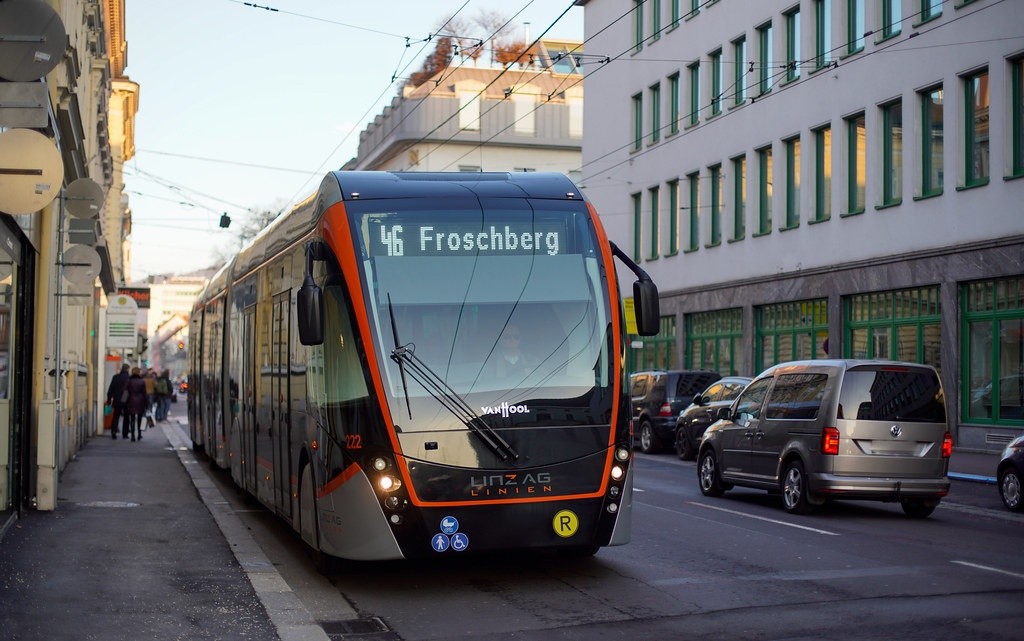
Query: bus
183,166,662,564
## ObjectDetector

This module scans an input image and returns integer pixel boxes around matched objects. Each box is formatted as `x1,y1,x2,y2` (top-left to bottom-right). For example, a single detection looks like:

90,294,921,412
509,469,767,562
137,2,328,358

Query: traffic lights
138,333,149,353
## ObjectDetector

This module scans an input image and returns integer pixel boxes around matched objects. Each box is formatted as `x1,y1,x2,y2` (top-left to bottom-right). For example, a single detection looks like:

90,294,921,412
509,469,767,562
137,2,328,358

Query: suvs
630,367,724,454
675,376,758,461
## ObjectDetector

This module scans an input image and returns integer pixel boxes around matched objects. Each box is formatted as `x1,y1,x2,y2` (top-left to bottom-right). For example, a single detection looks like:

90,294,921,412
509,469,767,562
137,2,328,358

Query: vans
695,357,954,519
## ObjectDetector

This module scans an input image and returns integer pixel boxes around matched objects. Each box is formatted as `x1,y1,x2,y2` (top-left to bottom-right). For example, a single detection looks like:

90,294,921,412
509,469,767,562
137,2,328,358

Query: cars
972,372,1024,407
995,434,1024,513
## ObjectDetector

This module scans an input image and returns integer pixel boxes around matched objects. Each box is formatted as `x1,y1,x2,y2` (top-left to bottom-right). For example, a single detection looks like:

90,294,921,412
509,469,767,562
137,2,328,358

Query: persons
107,364,173,442
474,322,544,380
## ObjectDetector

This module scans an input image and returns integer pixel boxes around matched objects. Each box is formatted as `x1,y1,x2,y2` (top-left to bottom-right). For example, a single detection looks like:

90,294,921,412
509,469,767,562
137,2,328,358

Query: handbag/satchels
140,415,147,431
147,411,155,428
104,403,112,416
171,394,177,403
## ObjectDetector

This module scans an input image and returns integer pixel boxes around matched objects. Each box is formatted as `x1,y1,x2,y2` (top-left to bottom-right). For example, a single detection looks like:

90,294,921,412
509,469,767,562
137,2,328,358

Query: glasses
502,333,521,339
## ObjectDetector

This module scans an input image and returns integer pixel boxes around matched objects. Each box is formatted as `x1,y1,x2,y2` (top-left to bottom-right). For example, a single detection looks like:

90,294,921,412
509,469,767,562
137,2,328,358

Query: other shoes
131,438,135,441
123,436,129,439
112,435,117,440
137,436,143,440
